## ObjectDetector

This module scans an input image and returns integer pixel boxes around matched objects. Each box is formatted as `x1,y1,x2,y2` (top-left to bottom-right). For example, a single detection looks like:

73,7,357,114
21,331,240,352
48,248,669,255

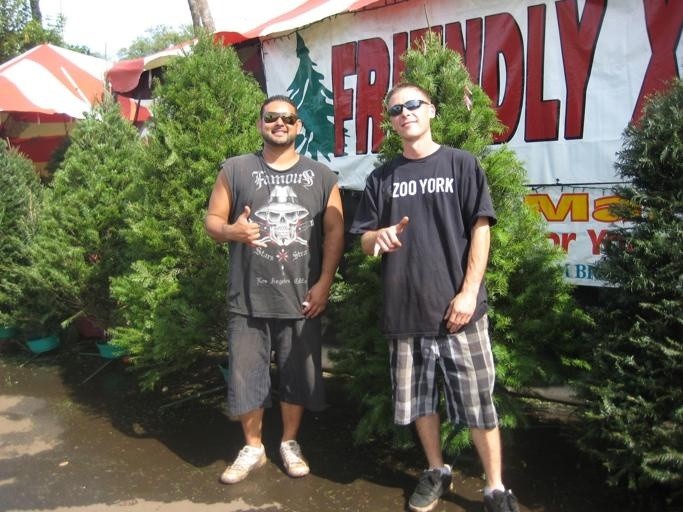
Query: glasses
387,100,429,116
264,112,297,124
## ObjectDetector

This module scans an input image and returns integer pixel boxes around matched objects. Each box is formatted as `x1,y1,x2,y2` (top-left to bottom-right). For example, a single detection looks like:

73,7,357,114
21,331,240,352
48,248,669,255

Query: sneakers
483,481,520,512
221,443,267,484
409,463,455,512
280,440,311,477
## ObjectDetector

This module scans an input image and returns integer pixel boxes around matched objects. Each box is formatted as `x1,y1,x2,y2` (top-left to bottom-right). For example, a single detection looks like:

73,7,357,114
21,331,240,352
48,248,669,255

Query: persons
201,94,345,485
349,82,526,512
